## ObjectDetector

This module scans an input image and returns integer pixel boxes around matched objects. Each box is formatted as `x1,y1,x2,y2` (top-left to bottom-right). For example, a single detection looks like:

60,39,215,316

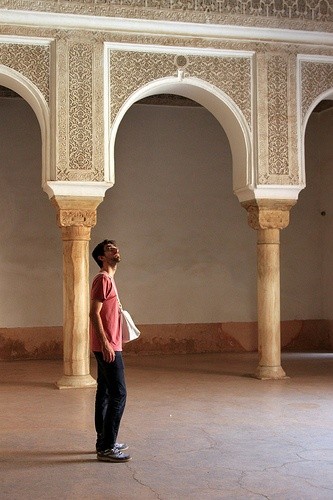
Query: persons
89,239,141,463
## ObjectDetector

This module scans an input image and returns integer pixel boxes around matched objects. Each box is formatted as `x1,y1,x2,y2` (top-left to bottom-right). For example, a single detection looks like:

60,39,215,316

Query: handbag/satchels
119,308,141,343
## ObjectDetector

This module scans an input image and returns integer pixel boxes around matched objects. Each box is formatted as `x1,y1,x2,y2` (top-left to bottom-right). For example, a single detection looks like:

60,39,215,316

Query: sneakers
97,448,132,462
114,442,129,450
